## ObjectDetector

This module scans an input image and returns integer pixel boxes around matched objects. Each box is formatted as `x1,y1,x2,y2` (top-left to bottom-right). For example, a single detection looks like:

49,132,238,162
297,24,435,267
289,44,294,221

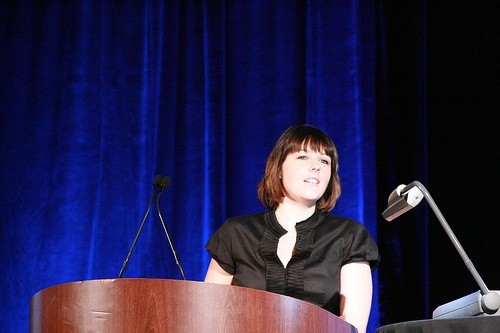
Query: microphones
117,173,187,280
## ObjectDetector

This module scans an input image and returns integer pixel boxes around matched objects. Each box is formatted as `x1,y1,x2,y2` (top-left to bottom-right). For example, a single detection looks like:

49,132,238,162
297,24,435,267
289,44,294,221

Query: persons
204,123,381,332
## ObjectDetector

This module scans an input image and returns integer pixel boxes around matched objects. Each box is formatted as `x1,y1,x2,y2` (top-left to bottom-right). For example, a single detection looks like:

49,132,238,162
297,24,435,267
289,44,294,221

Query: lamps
382,181,500,319
